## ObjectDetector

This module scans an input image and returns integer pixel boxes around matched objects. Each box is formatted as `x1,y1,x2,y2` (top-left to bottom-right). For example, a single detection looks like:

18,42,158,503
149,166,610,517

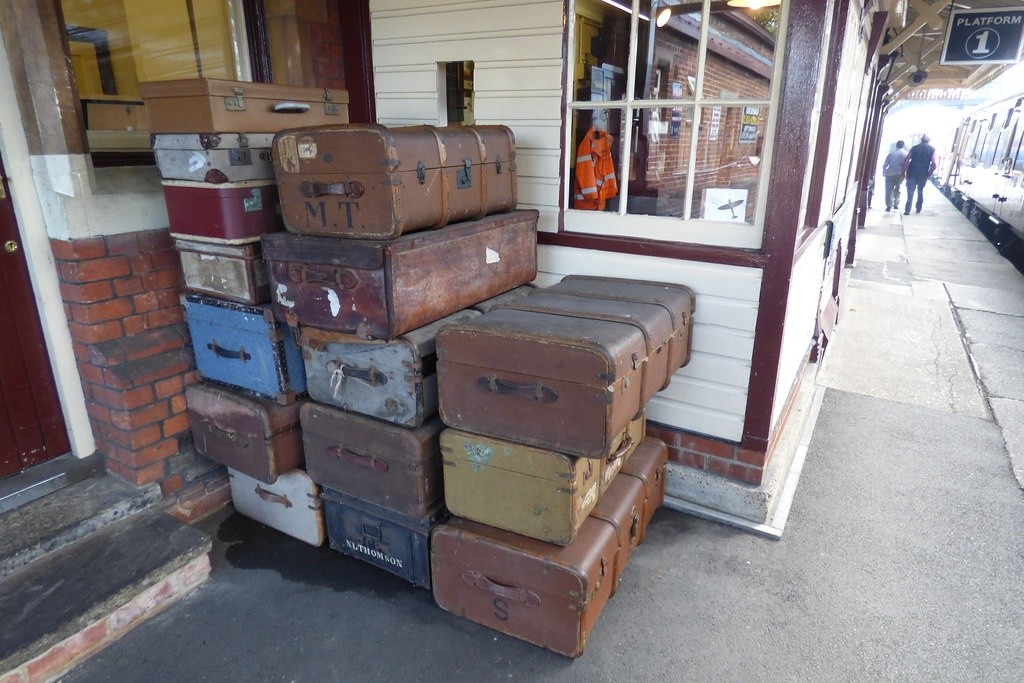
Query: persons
901,135,937,215
883,140,911,212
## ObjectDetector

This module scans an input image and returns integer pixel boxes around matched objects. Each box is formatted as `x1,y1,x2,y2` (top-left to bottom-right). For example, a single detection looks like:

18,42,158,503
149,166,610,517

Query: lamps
656,6,671,28
908,27,927,85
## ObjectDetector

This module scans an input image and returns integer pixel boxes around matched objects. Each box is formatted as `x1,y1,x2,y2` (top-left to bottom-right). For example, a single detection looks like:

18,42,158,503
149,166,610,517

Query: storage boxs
140,78,696,660
79,94,145,131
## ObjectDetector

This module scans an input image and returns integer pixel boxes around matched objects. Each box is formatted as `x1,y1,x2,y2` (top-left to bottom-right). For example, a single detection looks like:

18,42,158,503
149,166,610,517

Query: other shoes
885,207,890,211
894,205,898,209
916,209,920,213
904,212,909,215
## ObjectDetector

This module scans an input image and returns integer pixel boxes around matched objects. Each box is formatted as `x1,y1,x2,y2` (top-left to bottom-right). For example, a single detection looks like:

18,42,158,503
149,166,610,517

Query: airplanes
717,199,744,219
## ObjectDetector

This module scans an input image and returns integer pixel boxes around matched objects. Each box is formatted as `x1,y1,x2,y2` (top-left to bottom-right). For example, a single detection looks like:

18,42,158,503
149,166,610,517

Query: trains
933,91,1024,261
